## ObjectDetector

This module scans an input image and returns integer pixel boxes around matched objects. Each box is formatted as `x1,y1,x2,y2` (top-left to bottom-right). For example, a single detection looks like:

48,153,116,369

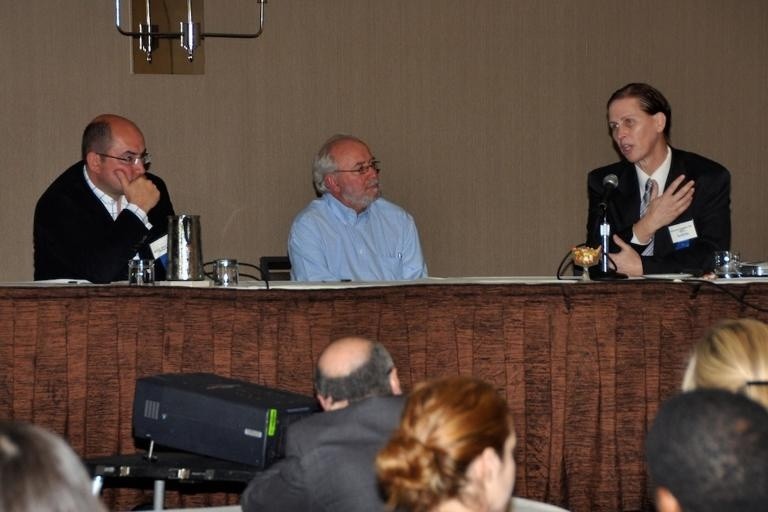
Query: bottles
166,214,206,281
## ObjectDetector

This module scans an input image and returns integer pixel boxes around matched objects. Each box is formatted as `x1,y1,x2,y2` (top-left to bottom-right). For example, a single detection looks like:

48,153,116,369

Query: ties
640,181,655,255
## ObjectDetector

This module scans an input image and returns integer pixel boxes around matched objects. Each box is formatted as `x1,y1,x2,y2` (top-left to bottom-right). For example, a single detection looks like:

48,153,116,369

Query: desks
1,275,765,510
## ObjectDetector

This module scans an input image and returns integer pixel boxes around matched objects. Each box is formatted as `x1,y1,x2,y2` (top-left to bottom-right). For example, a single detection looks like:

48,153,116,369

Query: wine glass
571,246,601,283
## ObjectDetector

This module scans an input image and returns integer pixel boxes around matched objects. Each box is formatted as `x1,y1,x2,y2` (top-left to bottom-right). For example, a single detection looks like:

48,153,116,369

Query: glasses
336,161,381,174
97,153,151,165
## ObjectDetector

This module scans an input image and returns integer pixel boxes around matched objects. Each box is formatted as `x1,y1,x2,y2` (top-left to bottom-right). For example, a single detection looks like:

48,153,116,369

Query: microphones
592,173,621,229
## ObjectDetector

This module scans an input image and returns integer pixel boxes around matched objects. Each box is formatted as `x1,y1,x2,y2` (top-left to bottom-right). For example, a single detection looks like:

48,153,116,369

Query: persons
3,416,107,511
644,386,767,511
287,134,430,282
373,376,567,511
33,114,176,280
680,316,767,411
587,83,731,277
240,335,408,511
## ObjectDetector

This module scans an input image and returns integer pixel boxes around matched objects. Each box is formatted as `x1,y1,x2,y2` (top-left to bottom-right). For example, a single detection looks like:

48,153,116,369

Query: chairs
256,252,292,282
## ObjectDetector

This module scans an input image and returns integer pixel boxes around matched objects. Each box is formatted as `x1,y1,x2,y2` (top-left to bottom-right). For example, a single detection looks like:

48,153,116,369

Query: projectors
131,370,324,471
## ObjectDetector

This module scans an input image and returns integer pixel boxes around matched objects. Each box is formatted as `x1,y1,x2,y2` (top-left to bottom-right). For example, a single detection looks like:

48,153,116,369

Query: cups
214,260,238,286
714,250,740,278
129,258,154,285
